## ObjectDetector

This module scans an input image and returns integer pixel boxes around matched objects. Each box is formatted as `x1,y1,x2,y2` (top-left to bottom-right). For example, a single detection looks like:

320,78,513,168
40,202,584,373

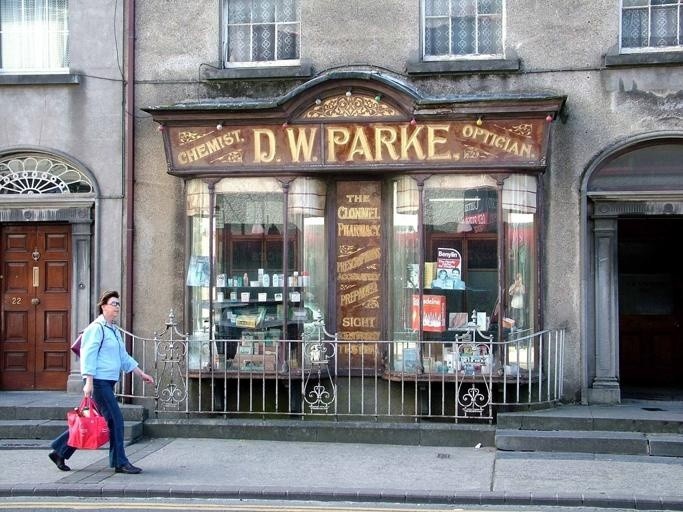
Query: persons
509,273,526,347
432,268,464,289
49,291,154,474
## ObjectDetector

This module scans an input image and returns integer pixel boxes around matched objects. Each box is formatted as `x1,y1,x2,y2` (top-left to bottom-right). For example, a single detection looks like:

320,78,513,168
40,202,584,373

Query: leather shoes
49,451,71,471
115,463,142,474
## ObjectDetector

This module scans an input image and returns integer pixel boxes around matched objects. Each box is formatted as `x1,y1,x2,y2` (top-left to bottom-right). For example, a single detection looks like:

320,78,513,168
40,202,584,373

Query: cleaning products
243,272,248,286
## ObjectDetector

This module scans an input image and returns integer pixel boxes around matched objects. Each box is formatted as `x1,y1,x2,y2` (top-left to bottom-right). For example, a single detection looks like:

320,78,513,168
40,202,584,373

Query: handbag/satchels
66,396,109,450
70,323,104,357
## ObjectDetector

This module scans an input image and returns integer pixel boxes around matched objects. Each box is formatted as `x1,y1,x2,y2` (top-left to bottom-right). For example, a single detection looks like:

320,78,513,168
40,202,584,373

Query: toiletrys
262,273,279,287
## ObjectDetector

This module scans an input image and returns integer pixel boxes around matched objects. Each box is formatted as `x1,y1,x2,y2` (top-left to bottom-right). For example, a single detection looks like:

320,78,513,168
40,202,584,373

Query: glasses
110,301,120,306
